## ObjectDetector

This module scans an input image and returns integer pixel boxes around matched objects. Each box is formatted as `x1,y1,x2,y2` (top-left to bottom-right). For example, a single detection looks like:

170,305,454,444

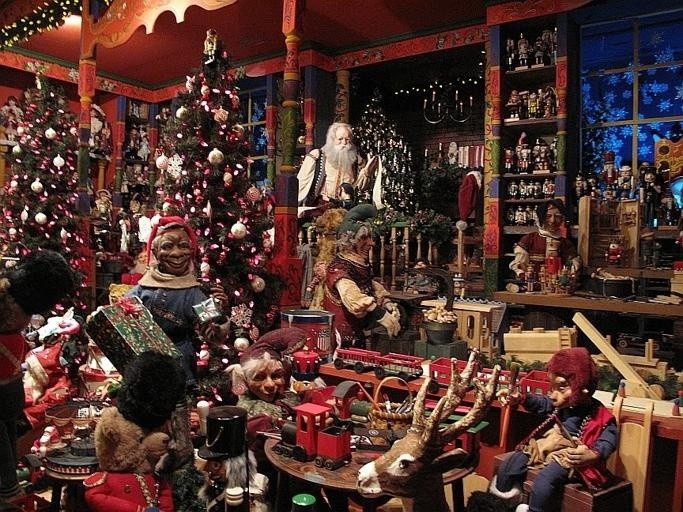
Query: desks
264,422,475,512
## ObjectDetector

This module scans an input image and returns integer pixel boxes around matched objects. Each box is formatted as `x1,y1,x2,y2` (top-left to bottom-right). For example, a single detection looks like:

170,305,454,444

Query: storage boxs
494,450,632,512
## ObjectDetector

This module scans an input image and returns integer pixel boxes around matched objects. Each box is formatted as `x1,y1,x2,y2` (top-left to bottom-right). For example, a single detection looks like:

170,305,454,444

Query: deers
355,347,501,512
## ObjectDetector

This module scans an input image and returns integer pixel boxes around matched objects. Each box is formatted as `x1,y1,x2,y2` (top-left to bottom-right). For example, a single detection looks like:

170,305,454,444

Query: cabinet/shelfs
503,64,557,282
491,217,682,377
